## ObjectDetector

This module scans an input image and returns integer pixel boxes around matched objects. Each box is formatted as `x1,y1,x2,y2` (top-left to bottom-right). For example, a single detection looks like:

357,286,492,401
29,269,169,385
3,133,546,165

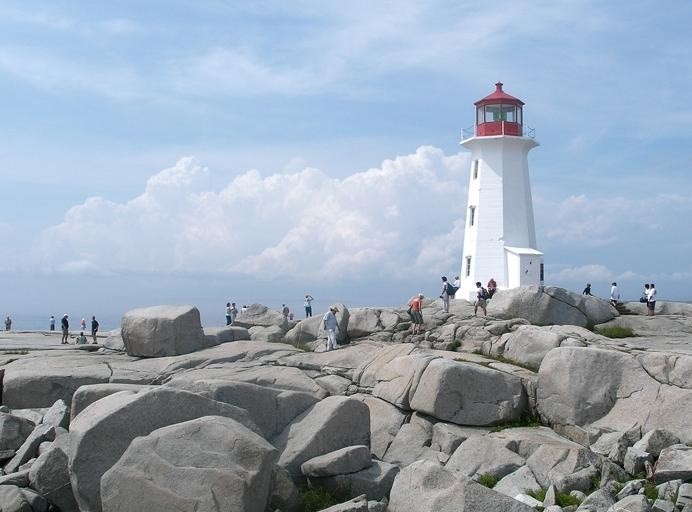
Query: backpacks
479,287,490,300
445,282,454,295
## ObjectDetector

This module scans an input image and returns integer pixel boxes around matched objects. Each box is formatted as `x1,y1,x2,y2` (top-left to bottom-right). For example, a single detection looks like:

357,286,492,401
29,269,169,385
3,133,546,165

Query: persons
231,303,238,322
583,283,593,296
226,303,233,325
282,304,289,317
609,283,619,308
487,279,496,299
450,276,460,299
241,305,247,312
643,284,650,316
441,277,449,313
648,284,656,316
4,317,11,329
50,314,99,344
304,295,313,318
474,282,487,317
407,293,424,335
323,307,340,351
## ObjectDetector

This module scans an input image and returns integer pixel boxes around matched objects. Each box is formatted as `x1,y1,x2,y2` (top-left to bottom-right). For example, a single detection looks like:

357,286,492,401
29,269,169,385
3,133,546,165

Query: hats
330,306,339,312
